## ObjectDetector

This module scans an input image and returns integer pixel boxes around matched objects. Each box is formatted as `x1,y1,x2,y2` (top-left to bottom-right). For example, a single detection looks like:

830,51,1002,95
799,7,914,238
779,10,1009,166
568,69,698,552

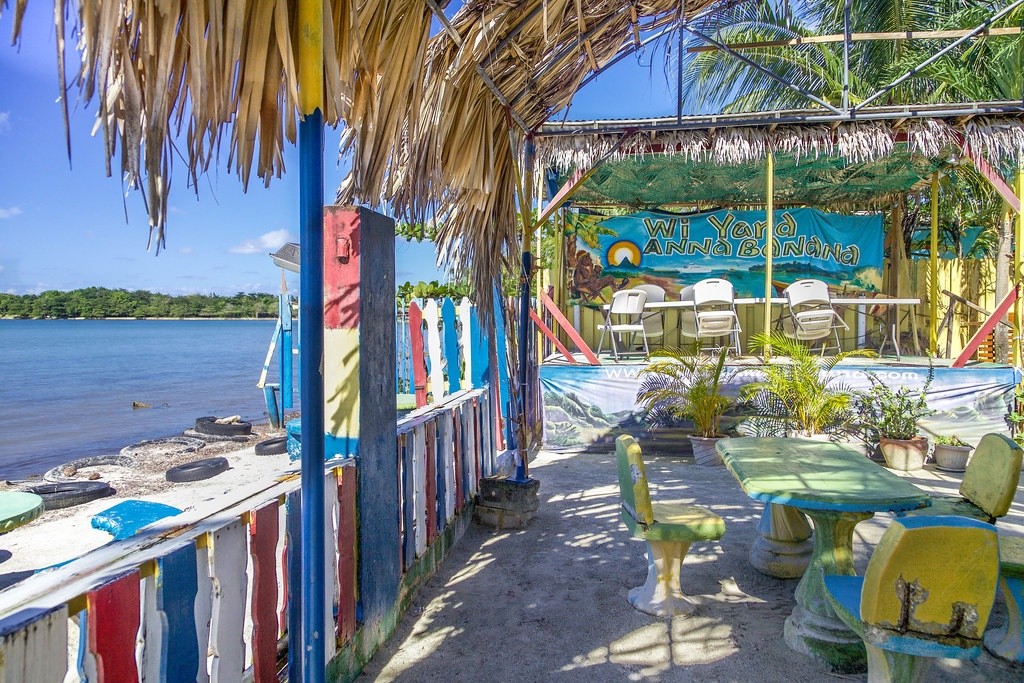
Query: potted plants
1008,383,1024,471
933,428,974,473
635,343,747,466
849,348,936,472
729,329,881,440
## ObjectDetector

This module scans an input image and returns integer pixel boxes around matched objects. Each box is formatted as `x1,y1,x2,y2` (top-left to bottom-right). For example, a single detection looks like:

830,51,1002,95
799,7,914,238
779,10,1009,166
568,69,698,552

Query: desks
712,436,933,672
602,298,921,361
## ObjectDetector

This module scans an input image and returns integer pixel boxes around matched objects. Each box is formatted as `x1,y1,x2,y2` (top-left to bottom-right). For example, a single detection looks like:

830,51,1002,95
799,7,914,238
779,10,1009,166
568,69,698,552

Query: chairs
692,278,742,358
890,433,1024,525
818,516,1001,683
771,279,850,357
676,285,715,352
625,283,666,354
596,289,651,363
613,433,725,620
983,535,1024,663
